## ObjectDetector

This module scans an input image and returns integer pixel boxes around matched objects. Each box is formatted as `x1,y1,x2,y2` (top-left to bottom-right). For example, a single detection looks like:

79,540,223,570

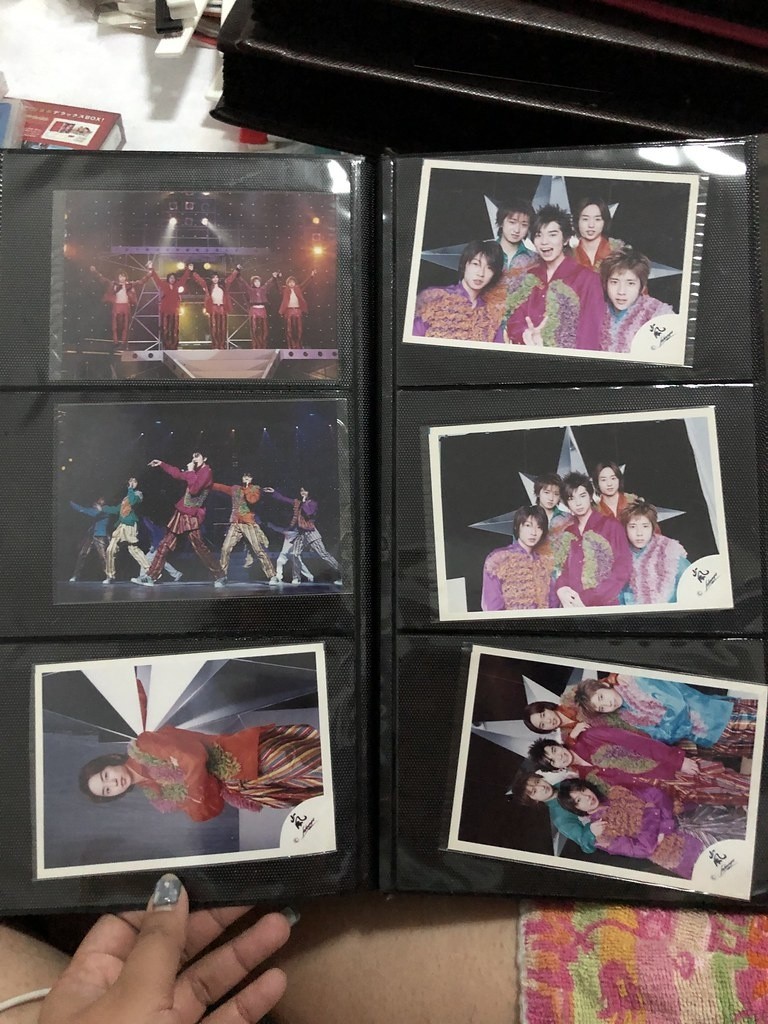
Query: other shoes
268,576,279,584
333,576,341,584
173,573,183,578
69,575,77,581
102,574,116,584
292,576,300,584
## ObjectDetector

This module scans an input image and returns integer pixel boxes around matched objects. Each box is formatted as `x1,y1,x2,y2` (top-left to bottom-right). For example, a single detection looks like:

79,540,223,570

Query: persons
480,460,700,611
64,448,345,586
78,721,323,823
409,194,680,353
504,672,761,882
84,260,311,355
3,871,301,1024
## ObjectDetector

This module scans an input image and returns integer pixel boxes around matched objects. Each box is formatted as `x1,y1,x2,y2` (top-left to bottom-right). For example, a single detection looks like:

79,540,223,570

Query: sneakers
129,574,153,587
215,576,229,587
